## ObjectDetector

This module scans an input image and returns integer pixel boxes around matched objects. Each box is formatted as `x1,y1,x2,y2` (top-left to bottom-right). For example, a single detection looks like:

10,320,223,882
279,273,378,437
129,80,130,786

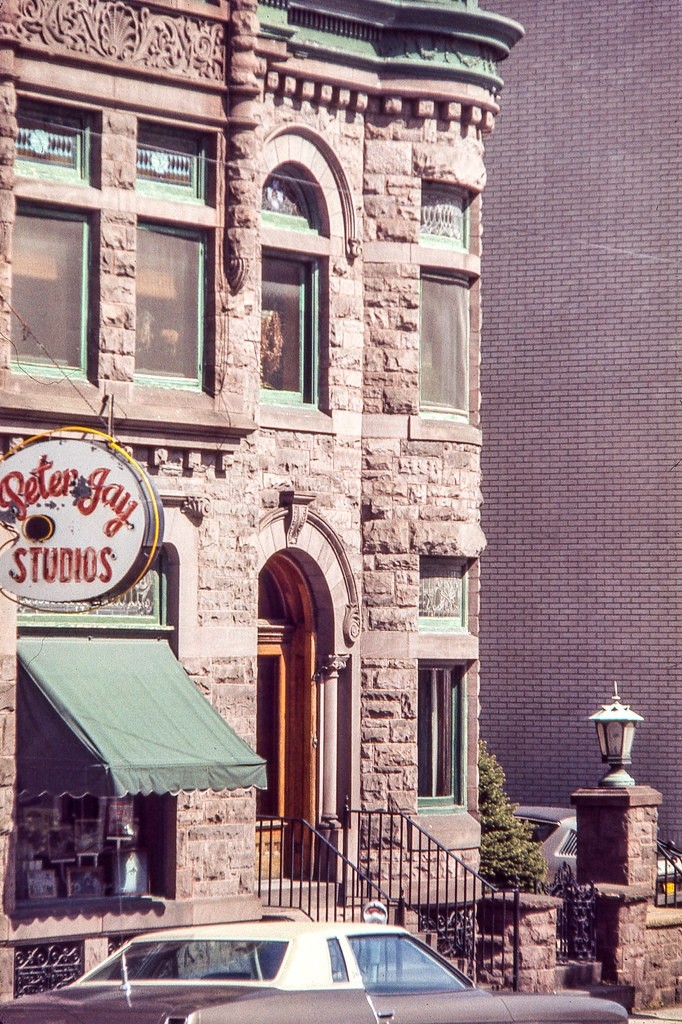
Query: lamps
586,681,647,788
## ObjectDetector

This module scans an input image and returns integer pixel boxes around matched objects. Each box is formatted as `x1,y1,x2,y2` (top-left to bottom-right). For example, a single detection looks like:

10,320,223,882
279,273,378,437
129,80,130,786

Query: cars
514,805,682,906
0,912,630,1023
